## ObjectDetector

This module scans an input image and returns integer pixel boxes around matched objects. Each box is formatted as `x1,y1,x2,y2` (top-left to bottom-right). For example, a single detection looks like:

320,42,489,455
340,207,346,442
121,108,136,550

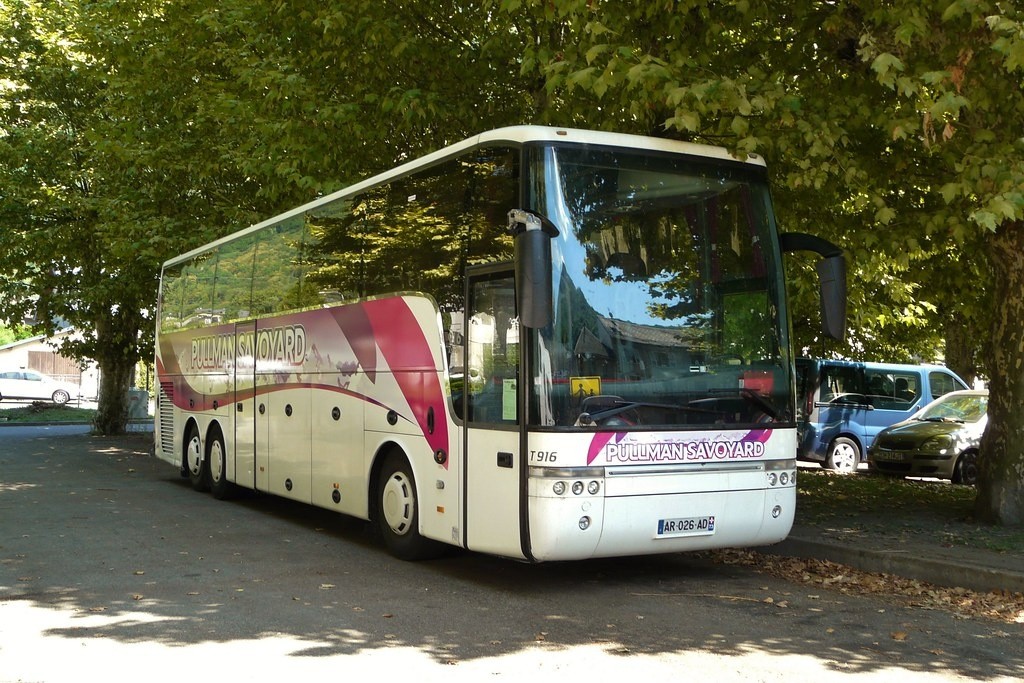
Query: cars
0,365,85,404
866,389,990,483
819,394,907,418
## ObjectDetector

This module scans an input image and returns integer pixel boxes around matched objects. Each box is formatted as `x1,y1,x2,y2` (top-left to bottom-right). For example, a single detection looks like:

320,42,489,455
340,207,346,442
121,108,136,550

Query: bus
155,126,849,564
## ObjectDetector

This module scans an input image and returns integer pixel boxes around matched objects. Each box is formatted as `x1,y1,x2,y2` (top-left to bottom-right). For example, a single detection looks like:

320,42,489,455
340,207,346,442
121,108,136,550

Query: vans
794,356,972,471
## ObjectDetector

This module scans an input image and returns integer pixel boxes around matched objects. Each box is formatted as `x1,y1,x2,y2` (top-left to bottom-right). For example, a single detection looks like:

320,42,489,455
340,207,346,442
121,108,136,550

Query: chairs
871,376,889,401
603,254,646,280
888,378,914,402
586,248,604,280
